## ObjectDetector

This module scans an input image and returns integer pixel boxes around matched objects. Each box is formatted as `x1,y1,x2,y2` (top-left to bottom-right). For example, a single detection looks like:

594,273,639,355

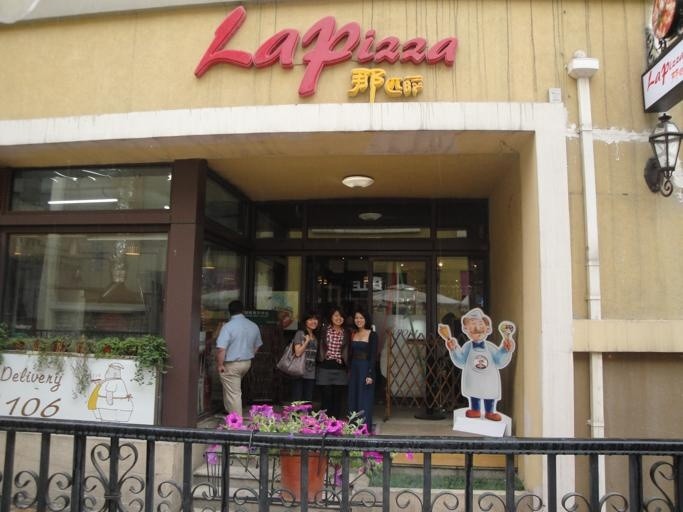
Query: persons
286,311,320,405
214,301,263,424
314,304,348,418
343,307,378,431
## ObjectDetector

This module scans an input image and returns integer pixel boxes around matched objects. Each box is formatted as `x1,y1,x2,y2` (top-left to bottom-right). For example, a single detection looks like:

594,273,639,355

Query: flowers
206,399,414,486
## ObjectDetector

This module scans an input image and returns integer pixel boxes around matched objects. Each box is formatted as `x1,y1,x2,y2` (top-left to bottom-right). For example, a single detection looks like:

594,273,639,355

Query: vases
279,448,330,504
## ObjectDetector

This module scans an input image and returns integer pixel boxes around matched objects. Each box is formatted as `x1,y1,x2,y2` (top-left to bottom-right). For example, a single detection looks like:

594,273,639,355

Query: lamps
645,113,682,198
342,173,376,190
358,210,383,223
47,197,120,206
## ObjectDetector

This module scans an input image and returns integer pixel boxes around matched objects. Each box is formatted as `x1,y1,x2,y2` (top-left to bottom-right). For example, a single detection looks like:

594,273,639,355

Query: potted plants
0,319,170,397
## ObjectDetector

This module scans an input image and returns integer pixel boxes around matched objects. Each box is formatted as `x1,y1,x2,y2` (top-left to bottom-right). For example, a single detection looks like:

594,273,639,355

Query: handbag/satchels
276,343,306,376
